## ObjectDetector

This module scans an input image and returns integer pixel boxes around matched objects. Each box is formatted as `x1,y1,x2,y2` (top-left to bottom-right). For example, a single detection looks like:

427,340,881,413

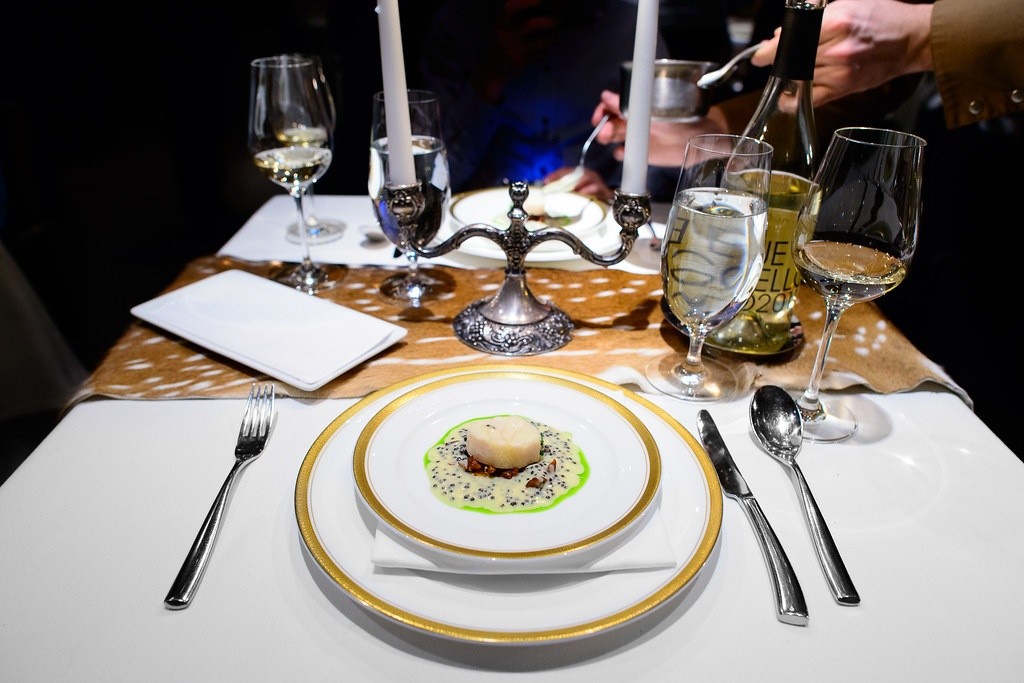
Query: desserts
423,414,589,513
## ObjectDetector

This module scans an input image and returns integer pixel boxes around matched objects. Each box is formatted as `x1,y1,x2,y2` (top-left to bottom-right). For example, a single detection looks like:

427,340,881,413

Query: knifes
695,410,809,627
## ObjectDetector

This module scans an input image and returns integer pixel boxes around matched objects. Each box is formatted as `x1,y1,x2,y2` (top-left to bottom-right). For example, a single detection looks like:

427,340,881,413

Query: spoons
750,385,860,606
533,112,621,201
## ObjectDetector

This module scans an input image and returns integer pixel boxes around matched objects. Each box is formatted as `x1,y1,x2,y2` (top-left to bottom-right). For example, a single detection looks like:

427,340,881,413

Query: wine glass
783,127,926,443
249,50,350,294
644,135,772,400
368,92,456,301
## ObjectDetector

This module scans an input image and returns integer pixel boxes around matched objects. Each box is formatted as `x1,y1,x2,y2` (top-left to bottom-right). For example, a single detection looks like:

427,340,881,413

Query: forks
164,383,274,610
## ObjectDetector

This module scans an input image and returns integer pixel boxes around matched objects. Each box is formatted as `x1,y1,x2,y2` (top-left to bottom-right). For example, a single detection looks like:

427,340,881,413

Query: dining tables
0,195,1024,683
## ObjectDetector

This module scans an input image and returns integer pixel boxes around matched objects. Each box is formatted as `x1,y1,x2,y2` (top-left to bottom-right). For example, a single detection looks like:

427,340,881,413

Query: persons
416,0,672,207
590,0,1023,171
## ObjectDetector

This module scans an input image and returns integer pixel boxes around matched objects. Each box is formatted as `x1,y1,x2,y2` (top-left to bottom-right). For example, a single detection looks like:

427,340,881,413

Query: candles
620,1,661,195
375,2,417,184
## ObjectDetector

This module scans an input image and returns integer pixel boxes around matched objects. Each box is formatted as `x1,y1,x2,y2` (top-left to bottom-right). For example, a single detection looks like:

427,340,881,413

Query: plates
293,365,724,646
352,372,661,561
130,270,407,392
447,185,610,241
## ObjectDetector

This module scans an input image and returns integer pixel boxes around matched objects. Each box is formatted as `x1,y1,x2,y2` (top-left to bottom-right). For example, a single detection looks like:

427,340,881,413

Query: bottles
709,1,829,362
